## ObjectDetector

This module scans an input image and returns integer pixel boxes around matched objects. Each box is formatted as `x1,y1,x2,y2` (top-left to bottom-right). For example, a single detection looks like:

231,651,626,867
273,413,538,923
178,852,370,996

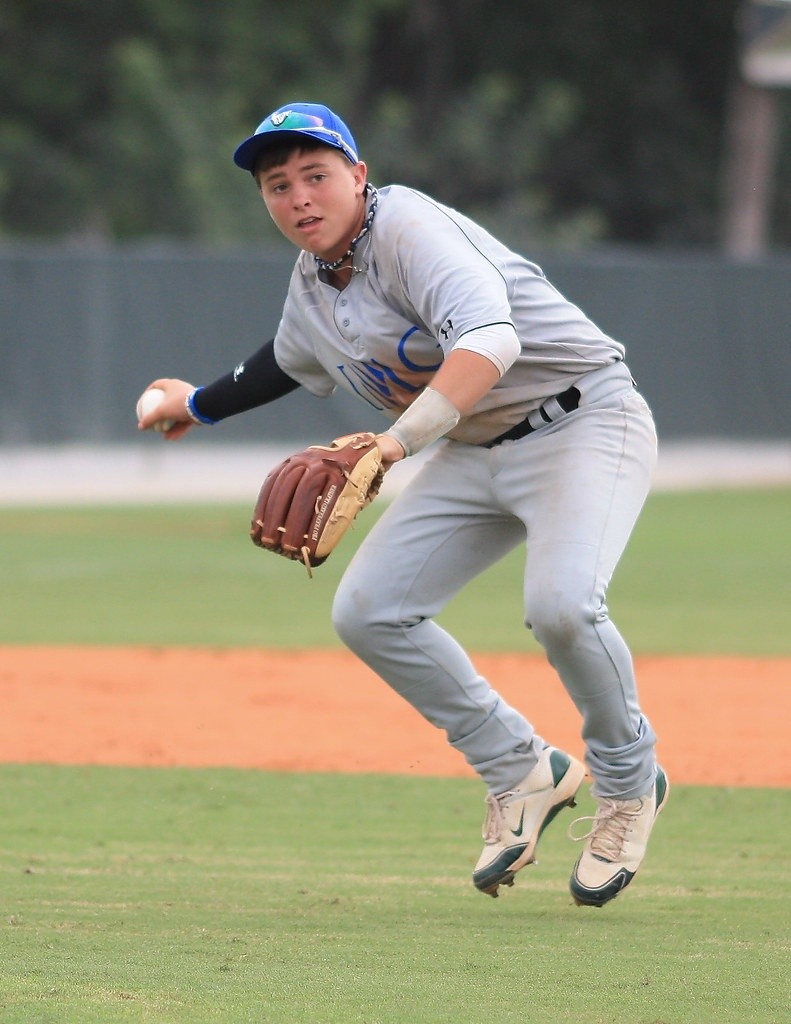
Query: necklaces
315,182,380,273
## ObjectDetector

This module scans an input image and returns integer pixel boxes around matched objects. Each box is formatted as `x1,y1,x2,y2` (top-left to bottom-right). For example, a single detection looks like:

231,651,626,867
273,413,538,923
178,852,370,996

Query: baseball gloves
247,434,389,564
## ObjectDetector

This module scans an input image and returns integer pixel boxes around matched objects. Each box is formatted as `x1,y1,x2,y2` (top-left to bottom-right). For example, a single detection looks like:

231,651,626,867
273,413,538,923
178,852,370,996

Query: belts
487,385,580,449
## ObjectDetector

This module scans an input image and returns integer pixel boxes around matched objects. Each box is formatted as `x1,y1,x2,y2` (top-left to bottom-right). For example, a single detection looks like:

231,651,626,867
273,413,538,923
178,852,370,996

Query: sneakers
472,742,589,897
568,764,670,908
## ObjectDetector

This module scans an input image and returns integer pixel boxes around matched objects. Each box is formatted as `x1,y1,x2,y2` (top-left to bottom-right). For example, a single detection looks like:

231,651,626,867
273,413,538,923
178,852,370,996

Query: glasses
251,110,358,163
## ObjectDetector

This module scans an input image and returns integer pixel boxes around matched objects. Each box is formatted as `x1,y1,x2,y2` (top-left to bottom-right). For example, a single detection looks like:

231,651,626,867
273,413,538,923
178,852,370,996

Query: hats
233,103,358,174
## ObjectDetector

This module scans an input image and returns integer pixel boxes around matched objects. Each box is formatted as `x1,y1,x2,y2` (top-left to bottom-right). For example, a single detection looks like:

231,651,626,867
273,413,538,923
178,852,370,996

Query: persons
135,102,669,909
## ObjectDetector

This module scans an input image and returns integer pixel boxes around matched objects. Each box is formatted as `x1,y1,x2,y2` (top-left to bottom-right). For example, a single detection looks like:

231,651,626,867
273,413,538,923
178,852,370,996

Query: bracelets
185,386,213,426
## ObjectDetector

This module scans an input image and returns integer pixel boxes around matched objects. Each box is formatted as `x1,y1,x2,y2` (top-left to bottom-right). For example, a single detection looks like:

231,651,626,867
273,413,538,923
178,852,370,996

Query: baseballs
135,387,182,431
